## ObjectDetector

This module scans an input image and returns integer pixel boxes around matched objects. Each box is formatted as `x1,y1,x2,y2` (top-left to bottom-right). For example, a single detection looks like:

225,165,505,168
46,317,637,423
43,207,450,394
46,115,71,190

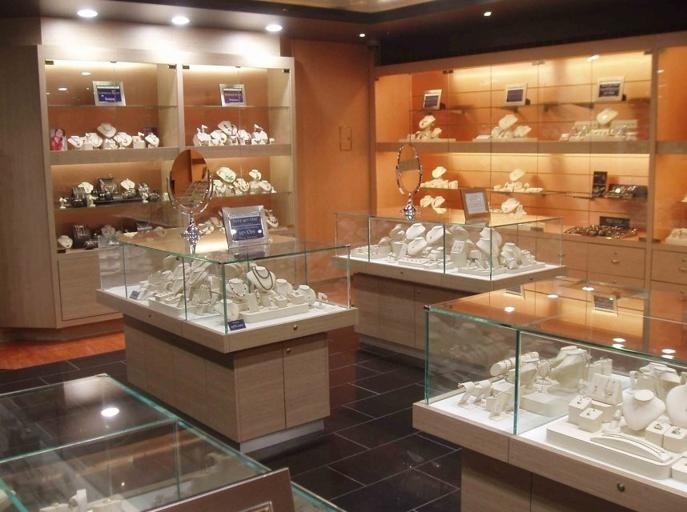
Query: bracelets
577,395,585,403
586,407,595,415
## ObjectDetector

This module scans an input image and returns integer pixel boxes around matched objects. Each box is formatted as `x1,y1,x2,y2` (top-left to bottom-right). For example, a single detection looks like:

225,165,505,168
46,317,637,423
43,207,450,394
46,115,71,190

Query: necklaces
406,226,520,262
60,237,71,247
592,373,605,393
66,122,273,150
207,166,278,224
433,198,439,206
603,377,616,398
502,346,587,372
421,118,428,126
601,110,614,120
81,182,90,191
166,255,273,298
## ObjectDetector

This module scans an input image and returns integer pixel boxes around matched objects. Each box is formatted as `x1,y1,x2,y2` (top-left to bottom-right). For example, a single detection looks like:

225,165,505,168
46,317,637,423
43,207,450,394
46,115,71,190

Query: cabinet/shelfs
177,60,292,289
44,54,182,325
373,47,655,360
647,40,686,369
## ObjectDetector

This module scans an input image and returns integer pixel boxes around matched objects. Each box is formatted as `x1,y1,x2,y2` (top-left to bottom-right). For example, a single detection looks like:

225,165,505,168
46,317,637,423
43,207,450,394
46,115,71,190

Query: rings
672,425,678,433
654,423,663,430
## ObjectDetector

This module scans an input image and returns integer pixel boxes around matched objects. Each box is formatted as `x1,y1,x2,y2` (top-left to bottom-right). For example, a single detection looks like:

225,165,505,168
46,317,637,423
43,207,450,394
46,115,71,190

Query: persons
48,128,65,152
